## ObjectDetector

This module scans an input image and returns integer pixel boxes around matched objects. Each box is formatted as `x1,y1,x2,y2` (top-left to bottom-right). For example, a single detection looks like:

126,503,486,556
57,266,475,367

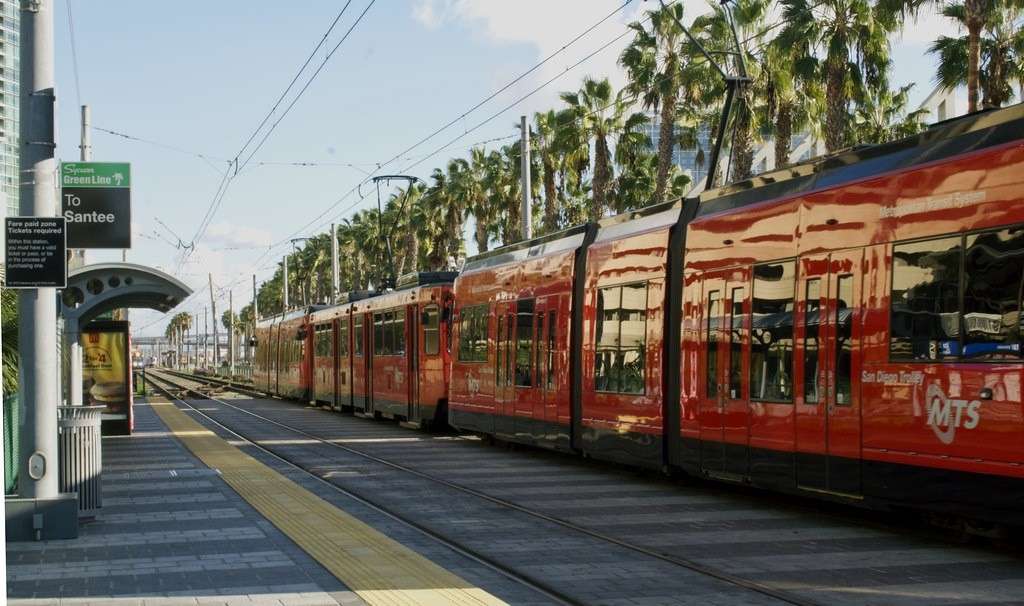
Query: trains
131,349,145,370
248,103,1024,538
